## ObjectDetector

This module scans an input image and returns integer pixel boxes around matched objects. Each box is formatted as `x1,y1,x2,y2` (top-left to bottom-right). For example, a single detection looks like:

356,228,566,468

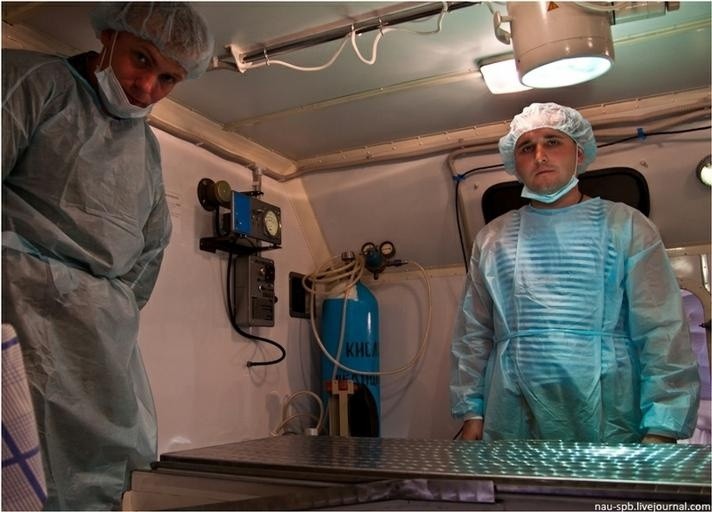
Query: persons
452,100,699,447
3,3,214,511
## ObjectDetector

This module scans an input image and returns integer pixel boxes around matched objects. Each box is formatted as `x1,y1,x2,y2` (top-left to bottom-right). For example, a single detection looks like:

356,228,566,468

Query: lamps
493,1,614,89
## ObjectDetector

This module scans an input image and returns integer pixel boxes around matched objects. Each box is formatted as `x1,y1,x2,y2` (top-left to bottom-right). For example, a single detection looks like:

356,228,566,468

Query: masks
521,144,579,204
94,31,153,119
